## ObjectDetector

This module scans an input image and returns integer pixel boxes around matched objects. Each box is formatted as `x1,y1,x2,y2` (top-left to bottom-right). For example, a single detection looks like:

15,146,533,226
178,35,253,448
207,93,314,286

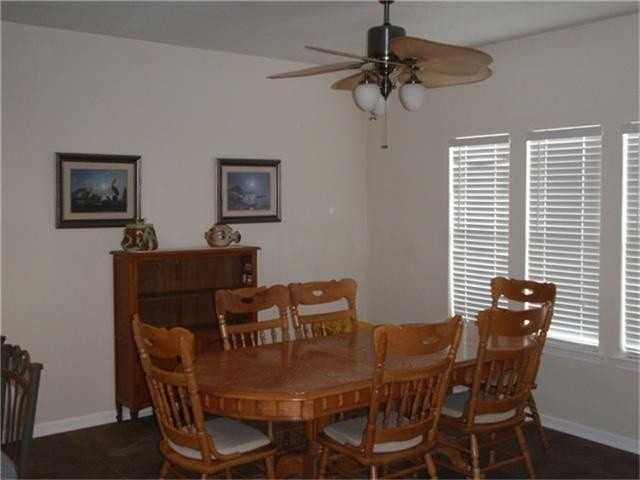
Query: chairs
452,269,556,464
2,336,45,479
433,298,552,479
213,279,295,458
128,313,277,480
286,278,363,420
315,312,462,479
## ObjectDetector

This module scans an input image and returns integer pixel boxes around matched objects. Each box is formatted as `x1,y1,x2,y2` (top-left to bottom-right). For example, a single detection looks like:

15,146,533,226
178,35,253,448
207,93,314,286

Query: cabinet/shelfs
109,246,263,429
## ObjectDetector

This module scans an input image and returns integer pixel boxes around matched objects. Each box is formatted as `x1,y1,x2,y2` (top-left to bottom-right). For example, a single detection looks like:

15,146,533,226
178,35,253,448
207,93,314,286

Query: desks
175,318,543,479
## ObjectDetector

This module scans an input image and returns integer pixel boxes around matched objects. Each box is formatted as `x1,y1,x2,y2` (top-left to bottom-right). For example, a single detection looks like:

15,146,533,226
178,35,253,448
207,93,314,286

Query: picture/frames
53,154,144,230
215,156,282,222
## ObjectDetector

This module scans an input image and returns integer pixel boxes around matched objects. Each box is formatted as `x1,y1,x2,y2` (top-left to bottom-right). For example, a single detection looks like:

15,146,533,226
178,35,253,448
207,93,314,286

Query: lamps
350,62,427,113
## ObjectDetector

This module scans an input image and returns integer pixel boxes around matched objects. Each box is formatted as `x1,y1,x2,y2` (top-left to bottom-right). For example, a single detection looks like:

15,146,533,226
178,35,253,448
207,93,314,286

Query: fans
262,0,494,86
